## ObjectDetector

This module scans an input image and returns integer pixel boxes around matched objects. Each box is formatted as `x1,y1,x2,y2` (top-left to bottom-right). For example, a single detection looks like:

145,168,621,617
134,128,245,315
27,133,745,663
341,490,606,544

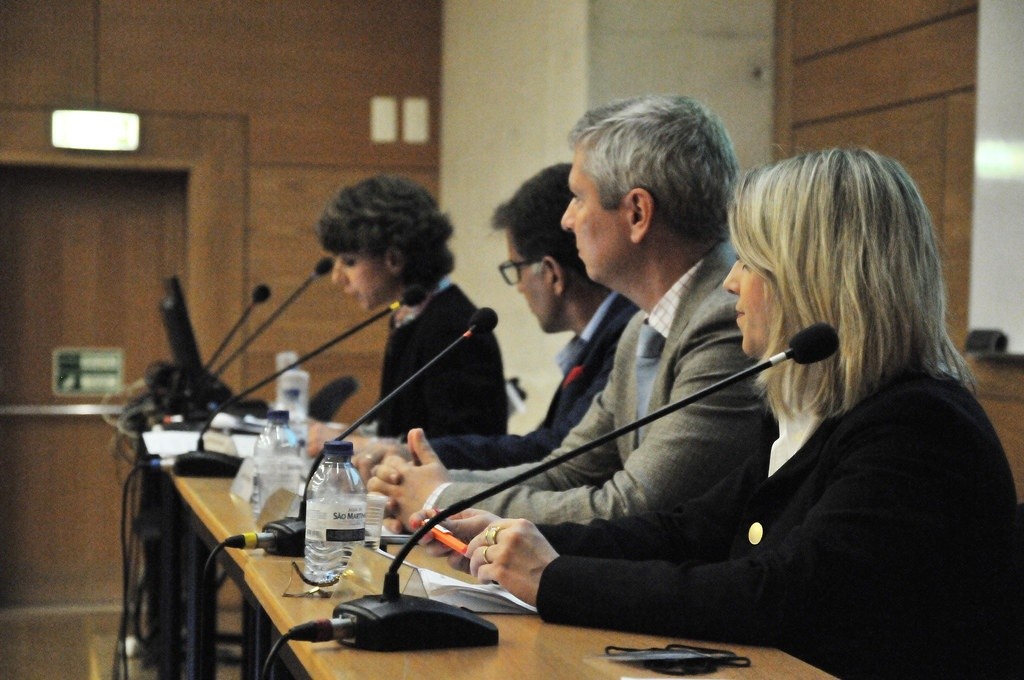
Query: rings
483,546,491,564
361,454,376,470
485,526,505,544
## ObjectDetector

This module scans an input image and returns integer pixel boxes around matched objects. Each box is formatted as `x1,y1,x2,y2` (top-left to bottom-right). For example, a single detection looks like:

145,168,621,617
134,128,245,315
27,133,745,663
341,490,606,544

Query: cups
364,493,388,551
258,461,301,533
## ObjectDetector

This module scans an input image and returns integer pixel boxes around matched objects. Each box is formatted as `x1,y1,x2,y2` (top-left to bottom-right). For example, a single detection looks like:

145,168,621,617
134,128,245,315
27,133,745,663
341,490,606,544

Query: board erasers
964,329,1006,354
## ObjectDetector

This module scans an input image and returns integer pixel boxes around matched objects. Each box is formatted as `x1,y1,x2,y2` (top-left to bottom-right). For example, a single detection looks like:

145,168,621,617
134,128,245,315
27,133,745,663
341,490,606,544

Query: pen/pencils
422,519,468,554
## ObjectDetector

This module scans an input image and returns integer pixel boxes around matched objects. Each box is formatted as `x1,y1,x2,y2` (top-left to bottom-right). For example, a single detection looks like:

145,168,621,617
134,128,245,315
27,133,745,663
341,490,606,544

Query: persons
410,145,1024,680
307,175,509,459
366,96,780,524
350,162,641,485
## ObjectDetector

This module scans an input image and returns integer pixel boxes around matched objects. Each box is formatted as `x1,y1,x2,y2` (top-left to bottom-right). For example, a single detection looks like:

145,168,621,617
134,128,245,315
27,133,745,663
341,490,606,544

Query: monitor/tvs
160,273,218,413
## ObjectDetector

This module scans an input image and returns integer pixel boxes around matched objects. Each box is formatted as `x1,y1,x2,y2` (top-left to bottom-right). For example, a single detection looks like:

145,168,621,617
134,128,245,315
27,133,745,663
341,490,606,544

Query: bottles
253,408,302,518
302,439,366,597
277,388,308,438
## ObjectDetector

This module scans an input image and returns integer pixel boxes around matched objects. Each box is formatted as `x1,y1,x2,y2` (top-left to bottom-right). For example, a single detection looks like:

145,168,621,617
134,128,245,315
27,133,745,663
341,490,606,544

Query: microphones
161,254,843,651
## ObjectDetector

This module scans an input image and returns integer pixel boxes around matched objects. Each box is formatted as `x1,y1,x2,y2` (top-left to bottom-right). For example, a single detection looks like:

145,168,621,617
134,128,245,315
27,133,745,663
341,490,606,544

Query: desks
140,440,840,680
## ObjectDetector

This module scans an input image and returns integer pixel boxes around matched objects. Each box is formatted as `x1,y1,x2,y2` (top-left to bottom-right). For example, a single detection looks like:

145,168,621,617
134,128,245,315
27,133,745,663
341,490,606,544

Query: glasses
498,257,543,286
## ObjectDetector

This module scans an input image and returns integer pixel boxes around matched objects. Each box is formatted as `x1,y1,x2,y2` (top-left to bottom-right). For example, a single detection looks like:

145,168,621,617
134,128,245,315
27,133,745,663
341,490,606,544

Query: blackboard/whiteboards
963,1,1024,361
